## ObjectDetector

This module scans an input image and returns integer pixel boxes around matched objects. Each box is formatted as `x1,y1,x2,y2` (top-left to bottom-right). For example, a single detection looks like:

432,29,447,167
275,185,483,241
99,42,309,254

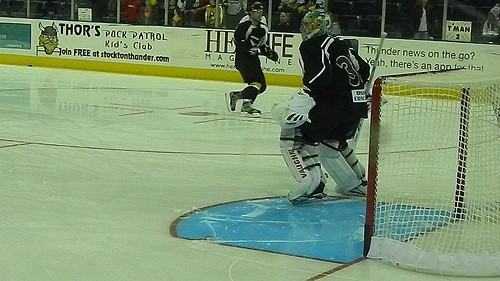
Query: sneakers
240,100,261,118
225,90,243,113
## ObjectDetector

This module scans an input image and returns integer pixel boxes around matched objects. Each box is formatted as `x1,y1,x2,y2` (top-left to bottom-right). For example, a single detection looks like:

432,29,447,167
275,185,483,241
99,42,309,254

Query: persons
224,2,278,117
0,0,267,29
278,0,500,42
279,6,372,204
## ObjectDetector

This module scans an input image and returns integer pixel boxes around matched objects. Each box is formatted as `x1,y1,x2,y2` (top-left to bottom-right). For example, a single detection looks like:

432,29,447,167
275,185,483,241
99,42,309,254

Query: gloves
250,27,266,40
261,44,279,62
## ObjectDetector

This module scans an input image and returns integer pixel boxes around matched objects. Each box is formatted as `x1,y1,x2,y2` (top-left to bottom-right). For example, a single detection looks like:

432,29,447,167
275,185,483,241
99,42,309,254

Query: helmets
299,8,332,42
245,1,264,13
